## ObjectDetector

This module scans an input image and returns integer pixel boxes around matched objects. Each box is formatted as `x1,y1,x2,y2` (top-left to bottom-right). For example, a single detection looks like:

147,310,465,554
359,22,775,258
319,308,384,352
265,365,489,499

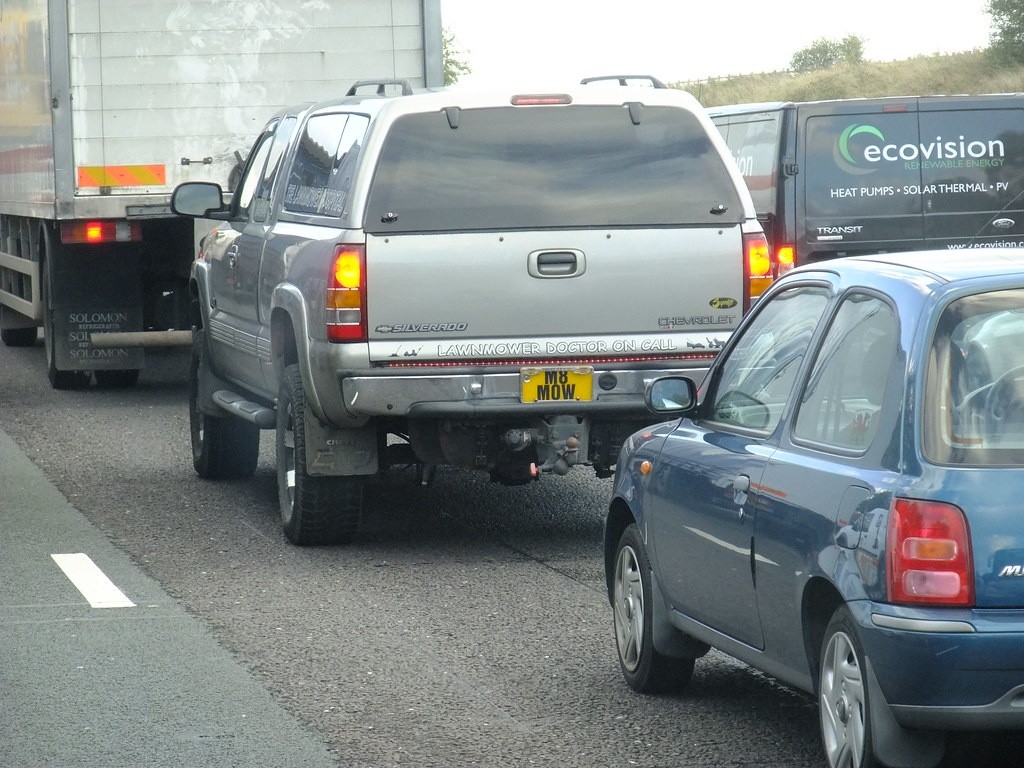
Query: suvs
169,73,778,546
704,91,1024,293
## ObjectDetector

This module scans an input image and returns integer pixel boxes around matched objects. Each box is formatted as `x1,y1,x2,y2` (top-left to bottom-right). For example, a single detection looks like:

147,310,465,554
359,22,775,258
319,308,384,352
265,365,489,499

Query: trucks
0,0,445,389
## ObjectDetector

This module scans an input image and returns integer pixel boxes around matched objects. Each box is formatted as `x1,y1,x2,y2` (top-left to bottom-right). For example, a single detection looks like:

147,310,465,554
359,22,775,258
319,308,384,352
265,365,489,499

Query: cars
604,246,1024,768
